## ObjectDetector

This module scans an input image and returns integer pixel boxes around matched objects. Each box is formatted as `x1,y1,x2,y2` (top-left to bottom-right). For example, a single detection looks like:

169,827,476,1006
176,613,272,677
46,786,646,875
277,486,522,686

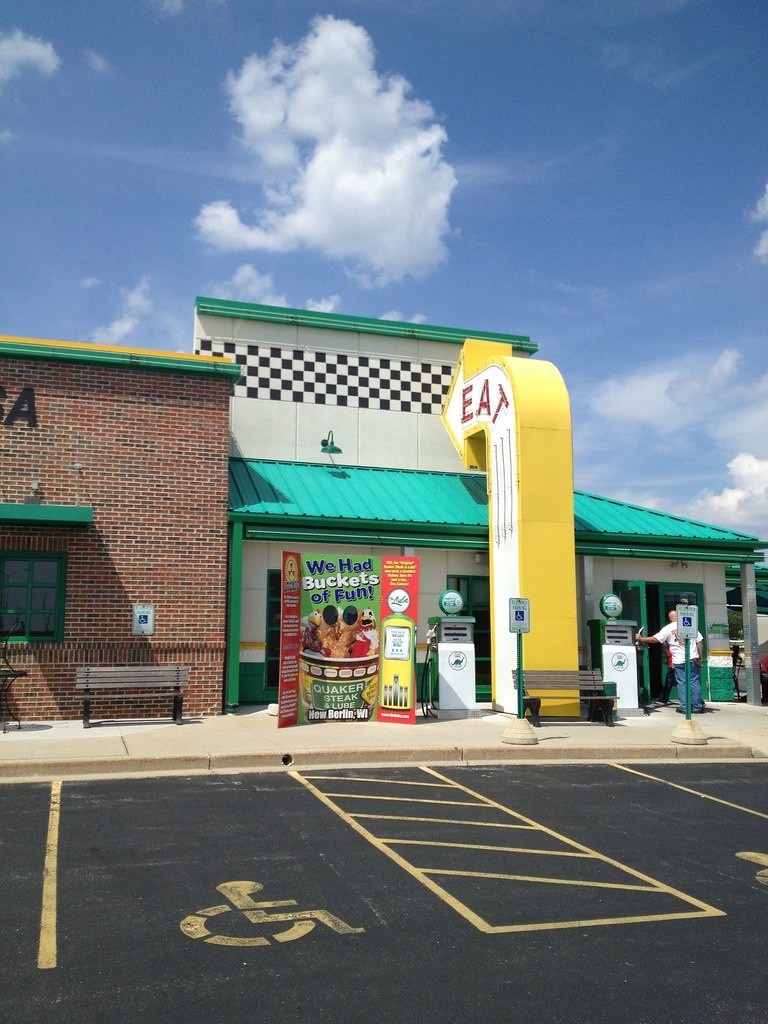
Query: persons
635,610,705,714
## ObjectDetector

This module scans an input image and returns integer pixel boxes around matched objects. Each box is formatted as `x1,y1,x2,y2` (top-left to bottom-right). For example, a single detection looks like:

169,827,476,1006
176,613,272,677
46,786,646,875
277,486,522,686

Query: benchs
512,668,612,727
74,666,189,728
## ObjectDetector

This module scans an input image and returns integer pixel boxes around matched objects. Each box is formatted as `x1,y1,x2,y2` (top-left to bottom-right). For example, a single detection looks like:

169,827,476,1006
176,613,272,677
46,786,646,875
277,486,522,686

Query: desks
0,618,27,733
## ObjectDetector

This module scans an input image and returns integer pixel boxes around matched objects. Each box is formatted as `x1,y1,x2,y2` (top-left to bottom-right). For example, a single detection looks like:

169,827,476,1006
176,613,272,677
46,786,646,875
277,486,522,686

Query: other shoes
676,707,687,714
654,697,668,705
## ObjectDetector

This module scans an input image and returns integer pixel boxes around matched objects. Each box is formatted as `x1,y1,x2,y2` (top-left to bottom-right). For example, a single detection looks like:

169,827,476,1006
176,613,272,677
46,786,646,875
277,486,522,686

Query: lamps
670,560,688,569
320,430,342,454
